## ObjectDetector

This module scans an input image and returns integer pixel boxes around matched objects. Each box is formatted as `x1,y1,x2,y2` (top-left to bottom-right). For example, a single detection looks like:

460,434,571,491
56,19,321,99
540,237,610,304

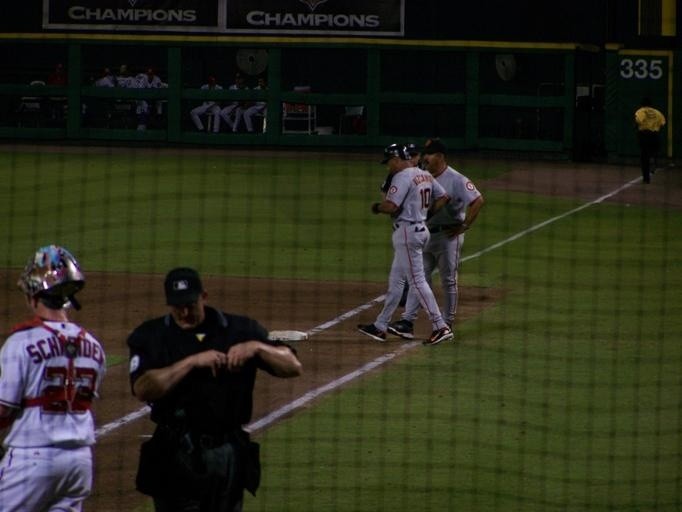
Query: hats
163,266,205,307
420,136,450,158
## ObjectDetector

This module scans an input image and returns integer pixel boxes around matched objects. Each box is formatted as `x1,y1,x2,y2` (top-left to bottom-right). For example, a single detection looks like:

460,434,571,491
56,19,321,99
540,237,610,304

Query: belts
428,222,467,234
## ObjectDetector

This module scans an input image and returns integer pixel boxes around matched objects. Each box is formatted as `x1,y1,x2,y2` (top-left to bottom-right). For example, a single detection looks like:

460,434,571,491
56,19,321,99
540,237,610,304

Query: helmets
15,242,88,305
380,142,423,166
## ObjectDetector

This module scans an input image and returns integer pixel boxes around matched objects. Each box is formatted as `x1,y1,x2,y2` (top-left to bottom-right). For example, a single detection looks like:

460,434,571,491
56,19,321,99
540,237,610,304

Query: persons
358,139,484,348
47,64,70,123
86,62,270,133
0,246,105,512
128,268,301,511
635,100,665,182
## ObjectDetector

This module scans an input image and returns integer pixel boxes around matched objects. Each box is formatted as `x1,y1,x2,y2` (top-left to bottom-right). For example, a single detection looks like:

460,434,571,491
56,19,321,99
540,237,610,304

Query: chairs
9,74,367,139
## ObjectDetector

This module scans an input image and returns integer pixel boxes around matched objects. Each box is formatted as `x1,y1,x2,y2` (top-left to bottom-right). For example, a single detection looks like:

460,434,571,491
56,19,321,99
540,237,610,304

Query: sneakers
641,165,657,186
356,323,388,343
421,322,455,346
387,318,416,341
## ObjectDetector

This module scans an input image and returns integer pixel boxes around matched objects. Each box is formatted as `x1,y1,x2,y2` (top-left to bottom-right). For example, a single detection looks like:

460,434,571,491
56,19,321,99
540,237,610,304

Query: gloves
371,202,382,215
